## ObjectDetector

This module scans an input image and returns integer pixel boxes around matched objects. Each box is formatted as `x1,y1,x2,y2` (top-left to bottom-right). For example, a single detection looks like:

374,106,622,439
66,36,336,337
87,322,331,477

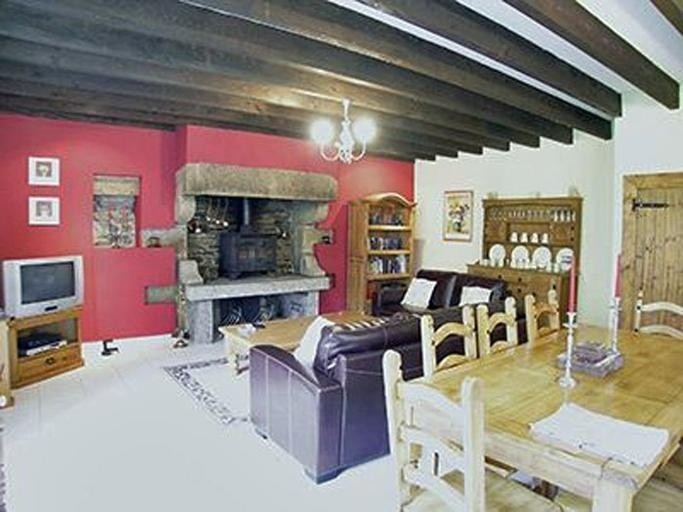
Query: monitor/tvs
1,253,85,318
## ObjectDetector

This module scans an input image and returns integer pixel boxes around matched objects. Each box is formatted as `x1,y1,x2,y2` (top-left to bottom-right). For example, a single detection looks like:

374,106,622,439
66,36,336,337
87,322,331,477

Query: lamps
312,98,376,165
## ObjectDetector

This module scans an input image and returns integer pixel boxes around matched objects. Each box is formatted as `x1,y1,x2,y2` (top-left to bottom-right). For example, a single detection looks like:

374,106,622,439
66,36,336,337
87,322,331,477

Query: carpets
162,355,250,426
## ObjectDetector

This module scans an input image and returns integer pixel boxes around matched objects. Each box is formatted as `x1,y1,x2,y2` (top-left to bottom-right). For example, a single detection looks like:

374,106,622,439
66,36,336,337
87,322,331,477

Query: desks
407,323,683,512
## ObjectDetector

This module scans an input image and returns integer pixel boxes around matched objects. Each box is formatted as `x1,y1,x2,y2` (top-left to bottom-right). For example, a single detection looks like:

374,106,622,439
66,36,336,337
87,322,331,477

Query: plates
510,245,529,261
533,247,552,268
488,243,506,261
555,248,574,272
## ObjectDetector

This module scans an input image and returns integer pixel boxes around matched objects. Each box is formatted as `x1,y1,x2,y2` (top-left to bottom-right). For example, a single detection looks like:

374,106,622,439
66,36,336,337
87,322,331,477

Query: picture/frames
27,194,61,227
26,156,61,188
442,190,474,242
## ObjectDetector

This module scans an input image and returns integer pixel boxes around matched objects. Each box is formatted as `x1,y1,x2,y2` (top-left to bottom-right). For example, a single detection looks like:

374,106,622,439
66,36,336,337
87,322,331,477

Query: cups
510,231,549,244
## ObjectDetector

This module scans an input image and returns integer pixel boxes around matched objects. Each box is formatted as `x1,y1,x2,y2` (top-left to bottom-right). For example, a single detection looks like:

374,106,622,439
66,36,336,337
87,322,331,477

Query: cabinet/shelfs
0,305,85,390
466,196,583,329
343,191,418,315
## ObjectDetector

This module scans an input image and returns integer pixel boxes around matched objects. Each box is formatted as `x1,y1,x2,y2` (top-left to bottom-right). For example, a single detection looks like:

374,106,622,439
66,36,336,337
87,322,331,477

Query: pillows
458,285,494,308
399,277,437,314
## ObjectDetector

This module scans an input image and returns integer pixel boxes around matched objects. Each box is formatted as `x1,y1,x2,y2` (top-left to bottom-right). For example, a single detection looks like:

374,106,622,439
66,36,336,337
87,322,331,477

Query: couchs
371,269,512,316
248,293,539,485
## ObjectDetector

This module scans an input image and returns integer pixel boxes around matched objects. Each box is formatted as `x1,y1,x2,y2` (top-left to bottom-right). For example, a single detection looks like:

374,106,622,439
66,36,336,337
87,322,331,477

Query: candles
614,253,622,297
567,256,575,312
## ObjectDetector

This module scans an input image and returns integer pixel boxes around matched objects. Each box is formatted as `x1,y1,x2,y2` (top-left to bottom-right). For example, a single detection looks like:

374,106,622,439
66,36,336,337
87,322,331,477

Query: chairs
420,290,561,480
380,348,562,512
633,292,683,341
554,442,683,511
